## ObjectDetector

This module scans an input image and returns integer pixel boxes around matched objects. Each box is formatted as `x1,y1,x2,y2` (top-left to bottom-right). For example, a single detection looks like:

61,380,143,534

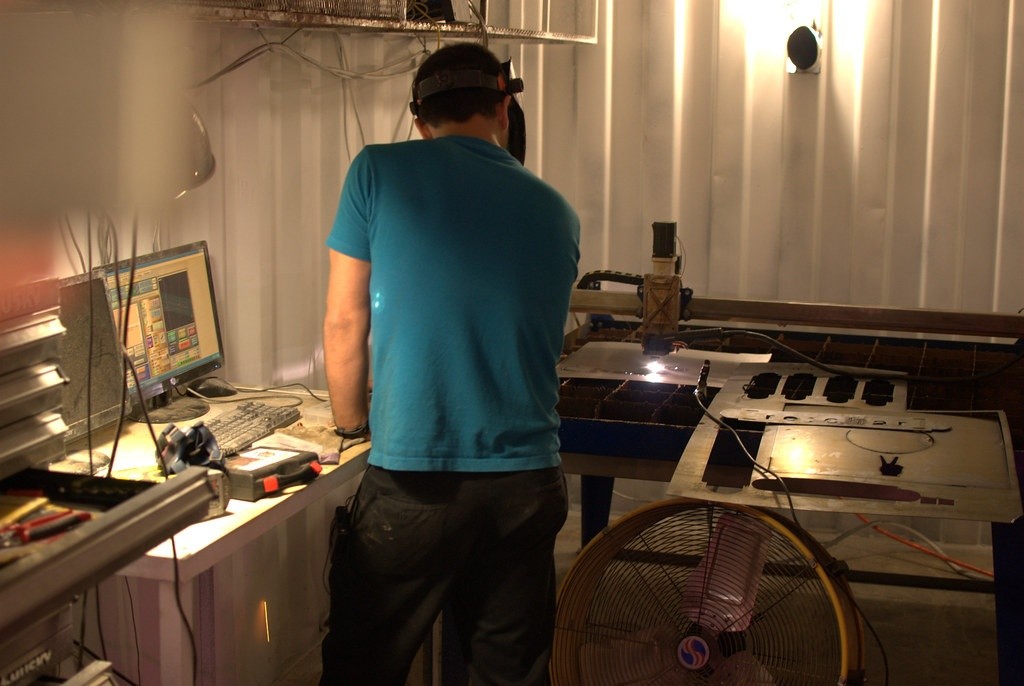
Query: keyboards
202,400,300,457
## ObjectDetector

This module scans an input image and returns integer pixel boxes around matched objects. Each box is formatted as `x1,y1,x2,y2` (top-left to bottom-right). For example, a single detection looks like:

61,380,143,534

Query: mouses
185,376,237,399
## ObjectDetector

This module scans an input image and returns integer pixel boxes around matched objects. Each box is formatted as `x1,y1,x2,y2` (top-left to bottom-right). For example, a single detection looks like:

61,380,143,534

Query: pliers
0,507,93,547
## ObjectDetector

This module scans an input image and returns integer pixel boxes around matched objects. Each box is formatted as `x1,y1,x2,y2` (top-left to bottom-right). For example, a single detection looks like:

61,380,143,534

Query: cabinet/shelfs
49,382,372,686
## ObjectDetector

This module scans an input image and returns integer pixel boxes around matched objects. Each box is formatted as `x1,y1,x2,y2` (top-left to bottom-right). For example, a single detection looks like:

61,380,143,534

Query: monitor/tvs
51,270,133,447
89,240,228,425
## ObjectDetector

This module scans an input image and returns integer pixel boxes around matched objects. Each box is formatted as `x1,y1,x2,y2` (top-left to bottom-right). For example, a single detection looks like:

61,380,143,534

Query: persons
318,42,580,686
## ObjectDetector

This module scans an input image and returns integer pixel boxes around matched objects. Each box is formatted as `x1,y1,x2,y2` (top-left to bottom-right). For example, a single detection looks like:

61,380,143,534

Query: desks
560,448,1024,686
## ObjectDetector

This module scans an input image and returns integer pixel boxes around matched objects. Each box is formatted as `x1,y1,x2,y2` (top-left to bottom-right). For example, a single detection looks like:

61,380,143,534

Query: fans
548,500,867,686
